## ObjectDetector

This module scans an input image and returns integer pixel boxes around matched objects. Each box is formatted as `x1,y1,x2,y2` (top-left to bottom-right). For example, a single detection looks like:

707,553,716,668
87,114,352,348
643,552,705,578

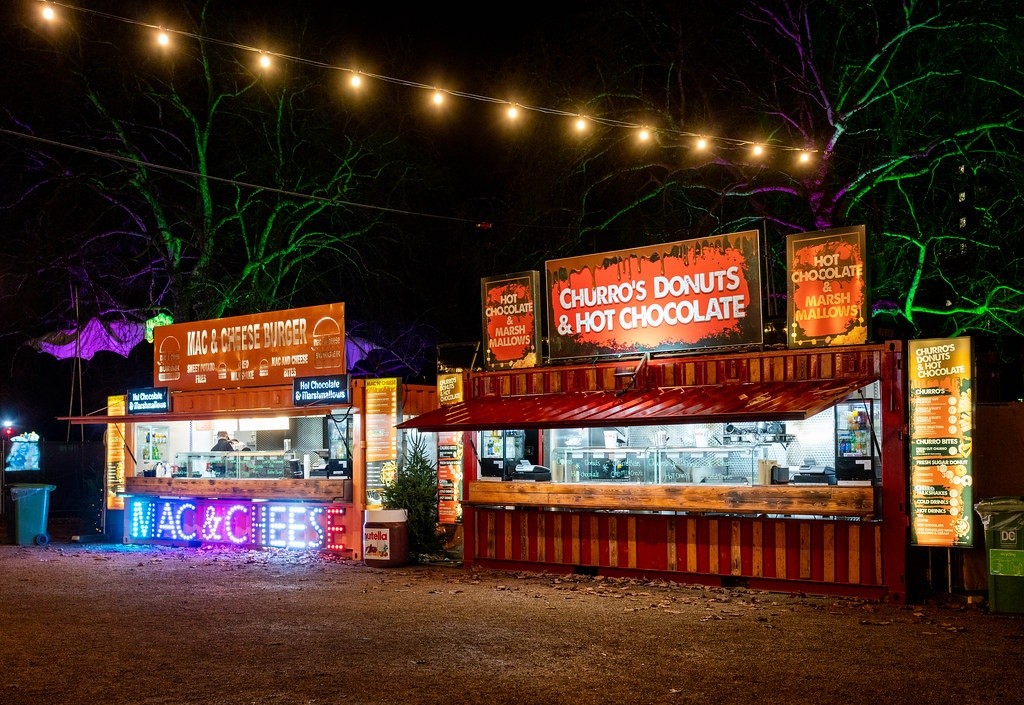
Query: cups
604,431,617,447
694,428,708,447
655,432,667,448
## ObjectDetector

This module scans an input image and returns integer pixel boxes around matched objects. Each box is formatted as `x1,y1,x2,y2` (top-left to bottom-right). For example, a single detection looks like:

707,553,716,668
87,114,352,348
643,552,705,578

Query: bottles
166,463,170,474
145,433,166,459
157,464,162,476
840,407,866,453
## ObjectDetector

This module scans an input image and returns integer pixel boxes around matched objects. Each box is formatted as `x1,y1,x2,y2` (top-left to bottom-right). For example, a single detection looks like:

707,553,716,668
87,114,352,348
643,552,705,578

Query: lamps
612,372,636,389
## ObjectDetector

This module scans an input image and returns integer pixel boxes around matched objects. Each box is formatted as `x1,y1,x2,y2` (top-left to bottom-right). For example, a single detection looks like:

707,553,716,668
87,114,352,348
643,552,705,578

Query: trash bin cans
974,497,1024,614
11,482,56,546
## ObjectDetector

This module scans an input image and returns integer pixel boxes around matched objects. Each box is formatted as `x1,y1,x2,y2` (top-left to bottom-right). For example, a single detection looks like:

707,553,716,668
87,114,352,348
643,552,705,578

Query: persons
210,431,251,477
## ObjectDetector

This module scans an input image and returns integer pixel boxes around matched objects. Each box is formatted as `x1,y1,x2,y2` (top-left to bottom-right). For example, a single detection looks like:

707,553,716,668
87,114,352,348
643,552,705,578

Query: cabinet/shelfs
137,425,169,469
